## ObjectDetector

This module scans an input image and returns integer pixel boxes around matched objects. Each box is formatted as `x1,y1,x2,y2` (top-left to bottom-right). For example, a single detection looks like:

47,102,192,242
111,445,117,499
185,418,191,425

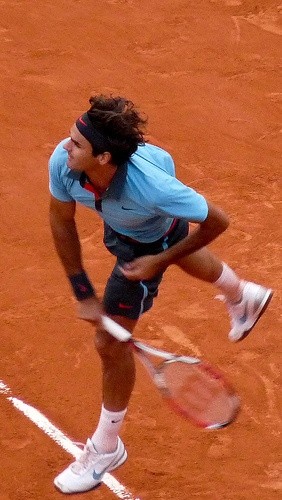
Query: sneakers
54,434,128,494
227,282,275,343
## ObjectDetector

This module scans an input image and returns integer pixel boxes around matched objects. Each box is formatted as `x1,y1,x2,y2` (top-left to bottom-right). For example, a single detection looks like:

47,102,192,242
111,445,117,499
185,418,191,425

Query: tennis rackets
101,315,240,430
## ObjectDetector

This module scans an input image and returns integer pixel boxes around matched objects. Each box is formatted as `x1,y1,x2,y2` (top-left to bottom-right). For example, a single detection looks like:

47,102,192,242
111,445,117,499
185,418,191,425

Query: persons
49,92,275,494
